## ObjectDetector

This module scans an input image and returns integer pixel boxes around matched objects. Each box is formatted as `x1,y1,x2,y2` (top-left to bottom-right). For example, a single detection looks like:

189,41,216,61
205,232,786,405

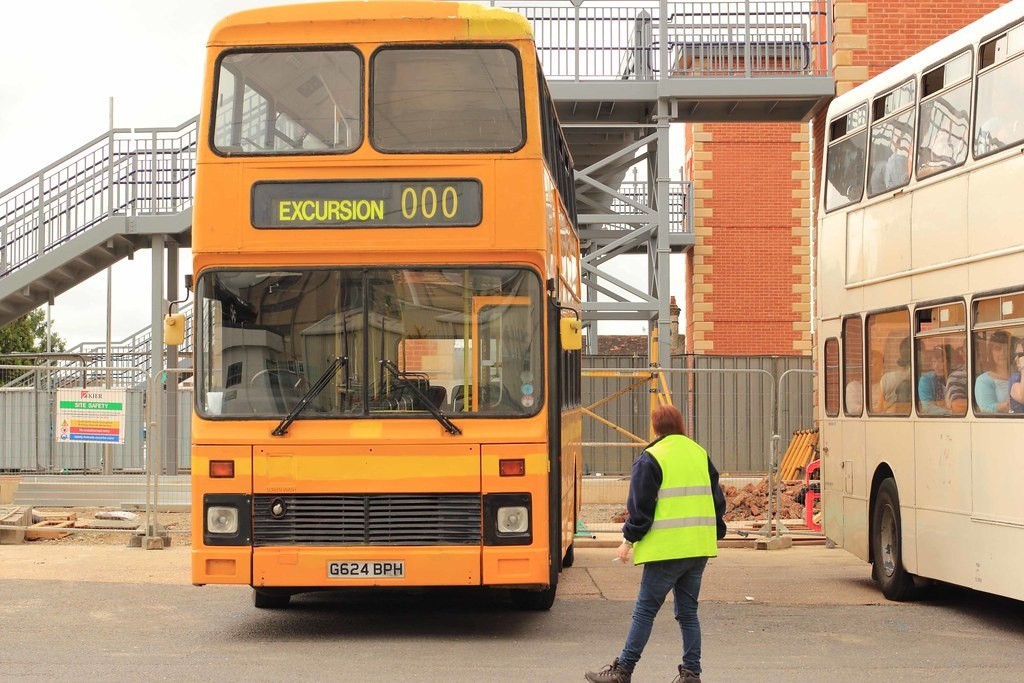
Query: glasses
1012,352,1024,358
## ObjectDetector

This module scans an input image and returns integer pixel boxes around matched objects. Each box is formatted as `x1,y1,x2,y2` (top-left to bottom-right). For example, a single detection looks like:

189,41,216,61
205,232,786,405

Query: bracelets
1018,364,1024,373
623,541,632,547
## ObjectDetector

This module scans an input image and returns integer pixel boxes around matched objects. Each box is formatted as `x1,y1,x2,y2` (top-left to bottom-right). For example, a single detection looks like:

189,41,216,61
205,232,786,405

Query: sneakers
671,664,701,683
585,657,633,683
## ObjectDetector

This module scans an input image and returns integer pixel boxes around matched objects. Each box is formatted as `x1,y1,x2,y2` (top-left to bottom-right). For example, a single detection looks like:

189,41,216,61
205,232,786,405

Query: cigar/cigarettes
613,557,621,561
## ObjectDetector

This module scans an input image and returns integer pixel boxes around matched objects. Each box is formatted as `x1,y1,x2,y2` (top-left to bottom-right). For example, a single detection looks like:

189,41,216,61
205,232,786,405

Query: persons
844,348,884,415
975,331,1014,413
585,404,727,683
918,344,954,413
1008,339,1024,415
947,338,988,415
881,336,926,415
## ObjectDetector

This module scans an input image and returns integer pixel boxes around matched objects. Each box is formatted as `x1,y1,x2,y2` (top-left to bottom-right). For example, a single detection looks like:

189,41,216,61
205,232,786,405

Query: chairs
352,372,521,414
846,371,1024,415
847,118,996,203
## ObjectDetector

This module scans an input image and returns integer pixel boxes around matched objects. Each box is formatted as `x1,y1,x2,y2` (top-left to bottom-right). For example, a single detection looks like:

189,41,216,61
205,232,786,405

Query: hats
897,336,926,367
